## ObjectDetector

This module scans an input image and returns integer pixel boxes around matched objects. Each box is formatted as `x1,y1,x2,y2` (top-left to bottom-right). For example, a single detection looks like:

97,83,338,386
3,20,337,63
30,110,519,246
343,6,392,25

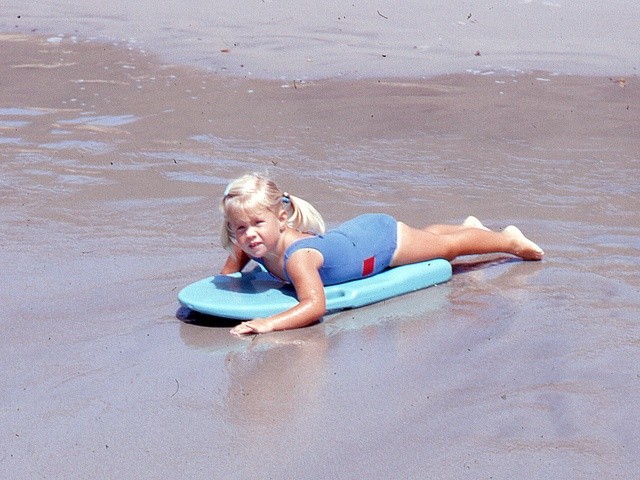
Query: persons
220,173,545,337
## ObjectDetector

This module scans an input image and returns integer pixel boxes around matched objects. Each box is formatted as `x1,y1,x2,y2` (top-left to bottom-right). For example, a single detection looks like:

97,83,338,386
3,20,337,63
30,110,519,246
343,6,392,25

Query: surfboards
177,256,453,321
